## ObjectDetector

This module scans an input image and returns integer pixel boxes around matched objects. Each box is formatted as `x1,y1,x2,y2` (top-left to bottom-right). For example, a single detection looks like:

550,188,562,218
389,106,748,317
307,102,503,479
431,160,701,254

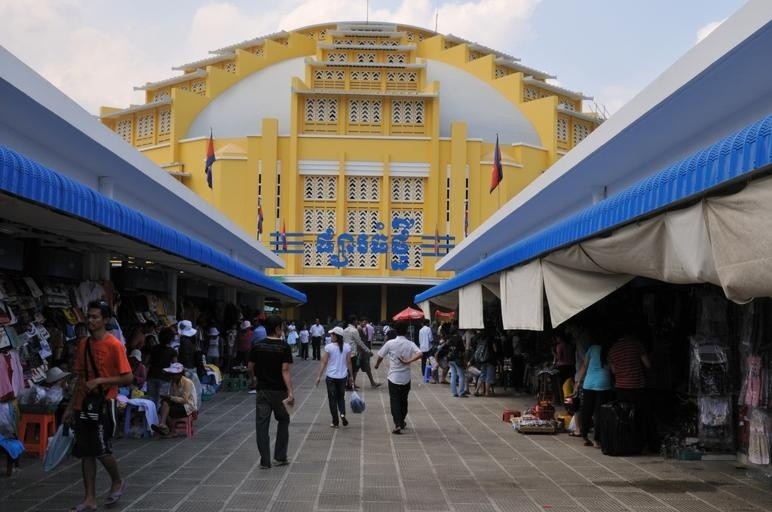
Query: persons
383,331,396,343
553,331,654,456
240,316,294,467
419,319,502,398
233,321,253,379
63,299,133,510
60,322,89,372
286,319,383,392
204,327,224,364
383,321,391,337
375,321,423,434
511,330,528,394
247,327,267,394
129,328,197,435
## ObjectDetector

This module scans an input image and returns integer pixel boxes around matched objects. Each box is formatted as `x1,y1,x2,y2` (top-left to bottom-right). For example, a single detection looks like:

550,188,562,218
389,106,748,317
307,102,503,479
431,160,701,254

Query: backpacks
474,336,490,362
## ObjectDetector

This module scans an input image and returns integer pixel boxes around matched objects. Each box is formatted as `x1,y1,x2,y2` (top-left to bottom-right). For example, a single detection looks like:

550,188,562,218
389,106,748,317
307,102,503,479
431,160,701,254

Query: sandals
151,423,169,435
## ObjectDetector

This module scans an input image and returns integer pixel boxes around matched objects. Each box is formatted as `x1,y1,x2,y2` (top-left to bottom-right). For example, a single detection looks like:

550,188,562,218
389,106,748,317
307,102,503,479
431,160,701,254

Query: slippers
68,503,98,512
104,479,126,504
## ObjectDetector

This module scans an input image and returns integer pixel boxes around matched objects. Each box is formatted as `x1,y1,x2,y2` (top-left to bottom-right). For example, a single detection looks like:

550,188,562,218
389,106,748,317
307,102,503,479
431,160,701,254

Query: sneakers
272,456,291,466
392,425,400,432
401,422,406,429
329,423,339,428
340,413,349,426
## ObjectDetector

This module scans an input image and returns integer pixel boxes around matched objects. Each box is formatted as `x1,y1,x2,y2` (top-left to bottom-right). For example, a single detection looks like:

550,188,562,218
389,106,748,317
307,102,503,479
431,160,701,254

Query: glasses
96,299,109,306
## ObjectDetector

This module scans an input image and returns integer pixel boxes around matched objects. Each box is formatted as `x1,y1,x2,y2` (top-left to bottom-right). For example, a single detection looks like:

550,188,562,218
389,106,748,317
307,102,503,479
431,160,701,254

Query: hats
129,349,143,362
163,362,184,373
207,327,220,336
178,319,198,337
241,320,251,329
328,326,344,337
46,366,72,383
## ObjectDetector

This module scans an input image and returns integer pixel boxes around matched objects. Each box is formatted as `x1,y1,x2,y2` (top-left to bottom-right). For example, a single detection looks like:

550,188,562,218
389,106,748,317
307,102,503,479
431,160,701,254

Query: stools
217,376,248,392
424,365,439,384
0,396,192,477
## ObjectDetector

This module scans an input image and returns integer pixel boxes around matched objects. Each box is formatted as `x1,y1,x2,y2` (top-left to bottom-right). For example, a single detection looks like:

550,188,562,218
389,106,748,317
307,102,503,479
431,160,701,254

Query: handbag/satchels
571,391,581,413
101,398,119,438
80,395,104,430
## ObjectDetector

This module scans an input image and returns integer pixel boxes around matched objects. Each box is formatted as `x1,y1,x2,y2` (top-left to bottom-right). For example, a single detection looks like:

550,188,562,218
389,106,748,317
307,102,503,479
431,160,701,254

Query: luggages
599,402,638,454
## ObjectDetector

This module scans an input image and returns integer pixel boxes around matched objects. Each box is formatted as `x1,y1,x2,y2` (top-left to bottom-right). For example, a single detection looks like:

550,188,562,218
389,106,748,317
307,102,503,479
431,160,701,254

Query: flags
490,136,503,194
435,226,440,253
281,222,287,250
205,133,216,188
258,207,263,234
